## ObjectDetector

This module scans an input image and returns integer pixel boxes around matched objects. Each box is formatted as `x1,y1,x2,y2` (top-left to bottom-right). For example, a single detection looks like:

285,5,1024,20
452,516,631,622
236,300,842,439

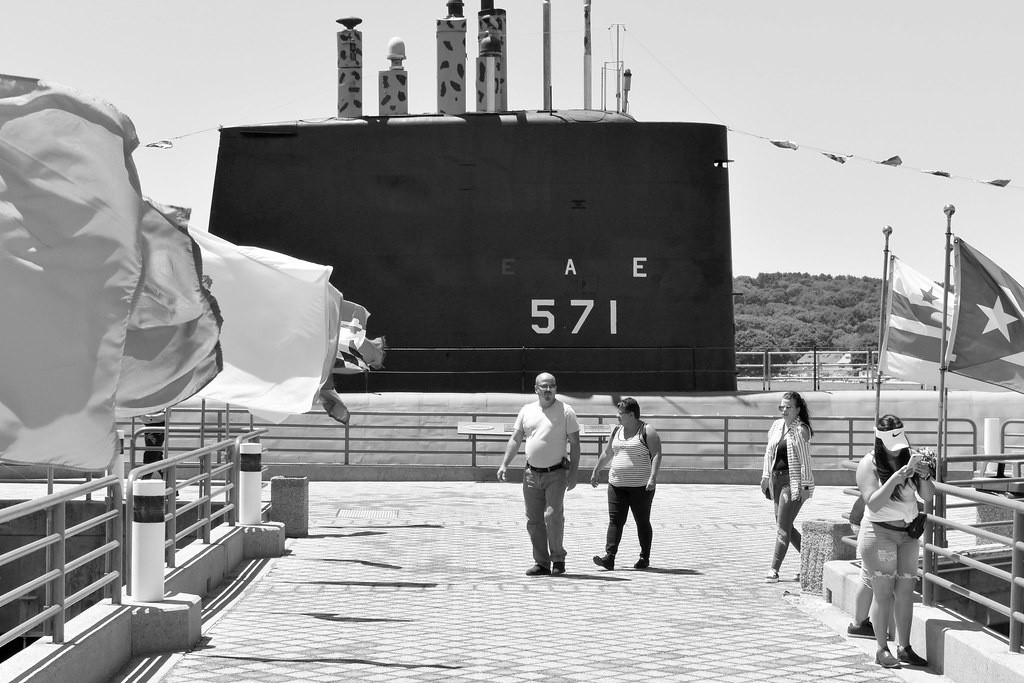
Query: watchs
919,474,931,481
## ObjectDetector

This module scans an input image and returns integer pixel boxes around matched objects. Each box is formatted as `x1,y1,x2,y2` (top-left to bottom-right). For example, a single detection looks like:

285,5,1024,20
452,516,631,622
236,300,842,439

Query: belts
525,462,564,472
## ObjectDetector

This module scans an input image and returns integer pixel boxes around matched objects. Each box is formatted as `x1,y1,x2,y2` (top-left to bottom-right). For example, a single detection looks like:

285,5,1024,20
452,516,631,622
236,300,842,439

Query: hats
873,426,911,452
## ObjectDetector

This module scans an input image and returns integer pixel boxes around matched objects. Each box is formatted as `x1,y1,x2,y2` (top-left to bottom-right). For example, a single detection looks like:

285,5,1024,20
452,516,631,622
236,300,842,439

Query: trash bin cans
972,459,1024,546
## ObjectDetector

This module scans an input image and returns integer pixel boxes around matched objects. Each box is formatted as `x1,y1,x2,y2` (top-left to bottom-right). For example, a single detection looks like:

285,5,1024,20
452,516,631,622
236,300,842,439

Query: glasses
779,406,795,411
616,409,630,414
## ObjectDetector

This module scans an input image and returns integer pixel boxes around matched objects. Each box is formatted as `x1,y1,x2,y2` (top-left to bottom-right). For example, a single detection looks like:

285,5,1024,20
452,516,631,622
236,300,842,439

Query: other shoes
634,557,650,568
765,568,779,583
552,562,565,575
526,564,551,575
846,617,890,640
875,646,901,667
897,645,928,666
592,555,615,570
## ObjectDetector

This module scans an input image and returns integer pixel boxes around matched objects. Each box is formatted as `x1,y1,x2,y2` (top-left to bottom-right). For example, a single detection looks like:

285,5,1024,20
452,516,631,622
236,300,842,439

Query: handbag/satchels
872,512,928,539
559,457,571,470
761,478,771,500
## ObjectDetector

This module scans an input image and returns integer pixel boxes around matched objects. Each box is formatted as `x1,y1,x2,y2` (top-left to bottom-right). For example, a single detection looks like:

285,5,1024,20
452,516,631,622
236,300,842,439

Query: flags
0,72,387,472
881,155,903,168
989,179,1011,187
922,169,953,179
946,237,1024,394
769,140,799,151
823,153,854,164
878,255,1014,392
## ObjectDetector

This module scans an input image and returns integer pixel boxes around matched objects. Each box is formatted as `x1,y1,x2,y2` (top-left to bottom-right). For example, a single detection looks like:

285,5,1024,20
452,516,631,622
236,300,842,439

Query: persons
496,372,581,575
590,397,662,570
847,414,936,668
760,392,815,583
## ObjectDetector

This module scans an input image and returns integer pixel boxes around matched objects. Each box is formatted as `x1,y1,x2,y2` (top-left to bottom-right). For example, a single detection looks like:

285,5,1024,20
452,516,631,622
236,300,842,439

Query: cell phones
904,454,922,477
